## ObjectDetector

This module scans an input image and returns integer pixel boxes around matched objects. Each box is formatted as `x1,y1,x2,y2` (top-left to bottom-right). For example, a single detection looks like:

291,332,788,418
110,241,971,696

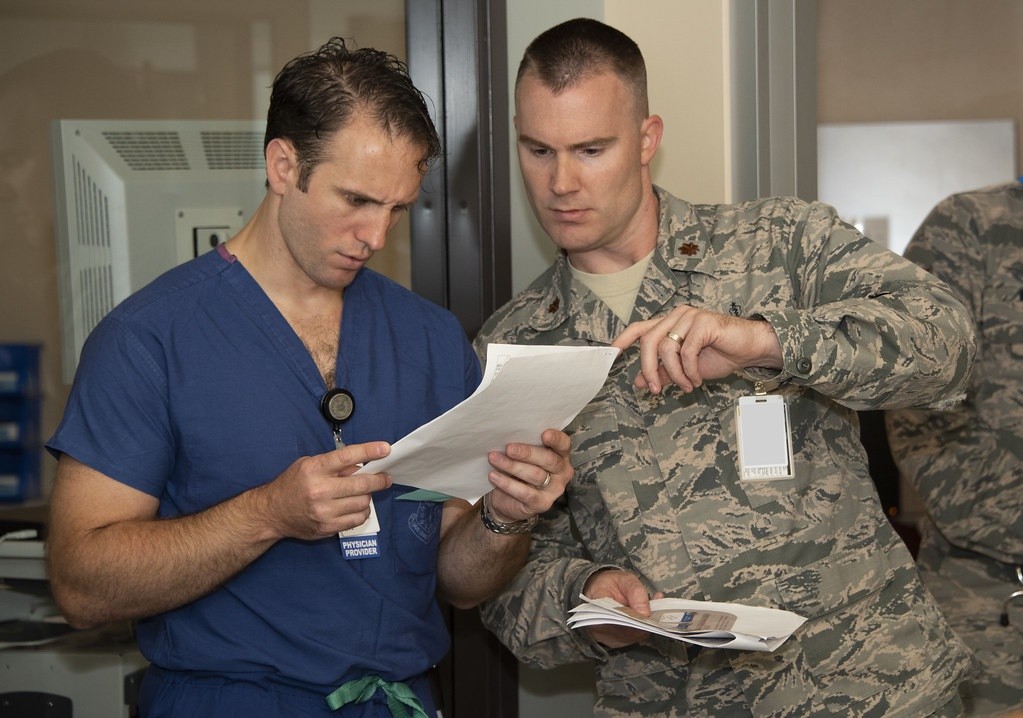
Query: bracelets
480,494,539,536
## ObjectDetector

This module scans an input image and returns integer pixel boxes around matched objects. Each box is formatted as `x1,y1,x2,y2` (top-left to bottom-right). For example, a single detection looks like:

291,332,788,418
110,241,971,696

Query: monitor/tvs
48,118,268,399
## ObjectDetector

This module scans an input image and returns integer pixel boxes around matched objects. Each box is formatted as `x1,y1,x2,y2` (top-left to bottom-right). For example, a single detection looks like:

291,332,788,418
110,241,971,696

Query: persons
471,15,974,718
881,179,1023,718
47,34,573,718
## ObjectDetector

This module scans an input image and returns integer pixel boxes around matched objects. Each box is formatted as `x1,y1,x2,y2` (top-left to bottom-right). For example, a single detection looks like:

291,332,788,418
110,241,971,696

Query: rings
667,332,684,346
533,471,551,490
